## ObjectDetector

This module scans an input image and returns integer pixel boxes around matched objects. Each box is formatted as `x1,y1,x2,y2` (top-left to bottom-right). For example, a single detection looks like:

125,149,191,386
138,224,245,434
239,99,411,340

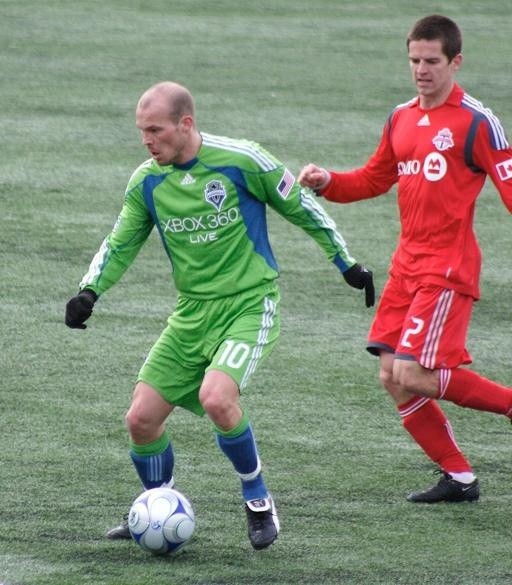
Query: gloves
65,287,98,329
342,262,375,307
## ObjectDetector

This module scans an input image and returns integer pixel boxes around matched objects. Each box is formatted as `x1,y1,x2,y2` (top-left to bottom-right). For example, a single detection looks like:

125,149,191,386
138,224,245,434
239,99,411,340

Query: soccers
127,487,195,555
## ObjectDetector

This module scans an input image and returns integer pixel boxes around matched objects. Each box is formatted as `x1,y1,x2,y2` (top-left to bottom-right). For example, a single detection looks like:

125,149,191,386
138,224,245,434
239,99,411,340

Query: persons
298,14,512,503
64,81,375,550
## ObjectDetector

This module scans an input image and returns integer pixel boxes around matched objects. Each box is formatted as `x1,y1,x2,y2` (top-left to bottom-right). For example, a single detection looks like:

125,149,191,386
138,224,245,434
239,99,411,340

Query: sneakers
245,491,280,550
106,512,132,539
406,468,480,502
